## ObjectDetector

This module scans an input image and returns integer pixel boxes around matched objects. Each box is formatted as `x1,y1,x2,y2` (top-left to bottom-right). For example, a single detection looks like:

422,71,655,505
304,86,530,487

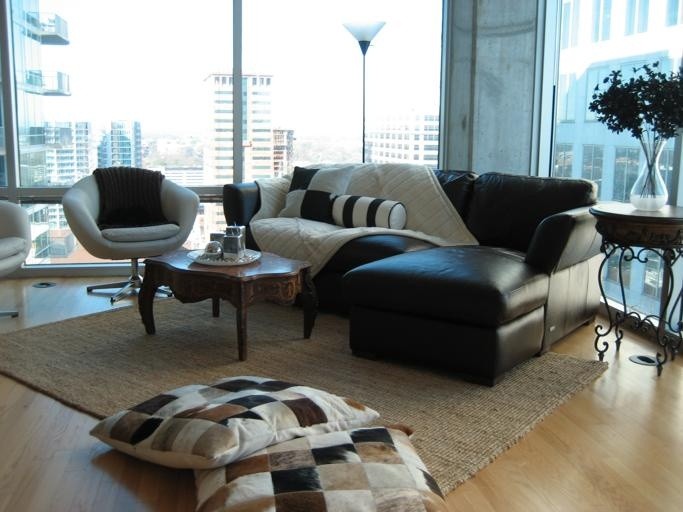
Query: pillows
278,164,355,221
189,425,447,511
332,193,406,232
88,369,382,474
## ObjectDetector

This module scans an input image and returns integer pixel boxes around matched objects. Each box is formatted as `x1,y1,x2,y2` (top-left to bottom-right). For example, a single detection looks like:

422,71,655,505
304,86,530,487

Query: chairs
61,167,200,306
0,195,34,321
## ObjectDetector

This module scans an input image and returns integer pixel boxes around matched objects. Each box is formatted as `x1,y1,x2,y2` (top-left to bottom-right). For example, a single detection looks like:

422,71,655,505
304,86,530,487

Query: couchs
223,160,610,387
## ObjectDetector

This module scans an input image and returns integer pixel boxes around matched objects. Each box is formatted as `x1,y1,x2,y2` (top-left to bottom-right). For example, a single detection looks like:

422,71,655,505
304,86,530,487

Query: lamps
342,19,386,163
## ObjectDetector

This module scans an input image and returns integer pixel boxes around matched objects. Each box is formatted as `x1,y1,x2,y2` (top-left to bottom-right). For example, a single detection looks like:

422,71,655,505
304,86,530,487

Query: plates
186,249,262,267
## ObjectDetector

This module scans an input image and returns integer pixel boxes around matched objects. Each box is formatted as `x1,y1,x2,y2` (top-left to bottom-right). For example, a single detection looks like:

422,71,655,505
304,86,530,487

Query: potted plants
586,45,683,214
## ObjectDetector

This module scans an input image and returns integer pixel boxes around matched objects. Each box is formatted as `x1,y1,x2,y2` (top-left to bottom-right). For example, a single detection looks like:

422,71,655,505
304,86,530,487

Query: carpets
1,284,605,494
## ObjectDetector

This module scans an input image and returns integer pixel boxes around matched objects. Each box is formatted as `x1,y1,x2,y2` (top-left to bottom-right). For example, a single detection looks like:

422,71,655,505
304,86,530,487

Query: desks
586,198,683,377
